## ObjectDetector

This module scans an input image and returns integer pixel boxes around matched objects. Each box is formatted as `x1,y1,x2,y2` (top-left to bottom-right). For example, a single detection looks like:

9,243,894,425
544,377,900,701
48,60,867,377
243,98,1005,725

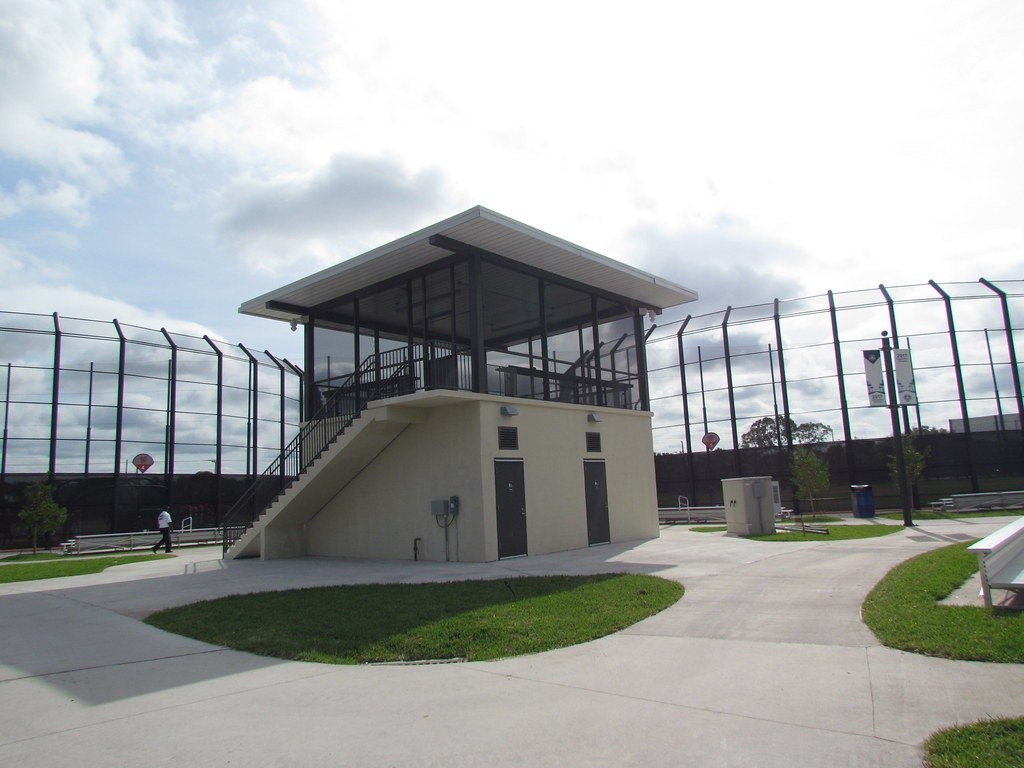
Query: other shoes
166,550,173,553
152,547,157,554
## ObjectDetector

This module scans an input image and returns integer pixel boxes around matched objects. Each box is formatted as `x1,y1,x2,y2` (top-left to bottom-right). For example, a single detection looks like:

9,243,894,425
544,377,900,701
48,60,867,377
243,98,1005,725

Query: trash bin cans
850,484,873,518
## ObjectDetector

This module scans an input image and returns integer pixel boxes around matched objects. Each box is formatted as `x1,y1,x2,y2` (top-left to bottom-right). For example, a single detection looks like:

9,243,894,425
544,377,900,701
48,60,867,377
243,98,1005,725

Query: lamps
500,406,519,416
588,414,603,421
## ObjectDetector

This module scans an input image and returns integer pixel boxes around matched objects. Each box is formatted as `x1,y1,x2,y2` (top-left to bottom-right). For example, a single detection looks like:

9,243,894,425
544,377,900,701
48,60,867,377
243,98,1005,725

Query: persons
152,505,174,554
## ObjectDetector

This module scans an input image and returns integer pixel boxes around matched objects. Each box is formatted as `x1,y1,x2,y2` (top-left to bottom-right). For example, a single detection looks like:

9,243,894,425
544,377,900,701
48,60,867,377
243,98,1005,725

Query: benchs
60,526,245,557
967,516,1024,608
927,490,1024,509
658,506,795,523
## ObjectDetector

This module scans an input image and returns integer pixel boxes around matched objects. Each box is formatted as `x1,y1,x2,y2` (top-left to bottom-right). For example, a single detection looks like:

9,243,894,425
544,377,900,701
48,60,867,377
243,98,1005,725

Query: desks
495,365,634,409
322,375,421,418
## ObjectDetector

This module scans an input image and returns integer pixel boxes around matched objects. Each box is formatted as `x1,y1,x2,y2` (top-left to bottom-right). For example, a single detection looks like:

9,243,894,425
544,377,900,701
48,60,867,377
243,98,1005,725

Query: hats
161,505,170,510
